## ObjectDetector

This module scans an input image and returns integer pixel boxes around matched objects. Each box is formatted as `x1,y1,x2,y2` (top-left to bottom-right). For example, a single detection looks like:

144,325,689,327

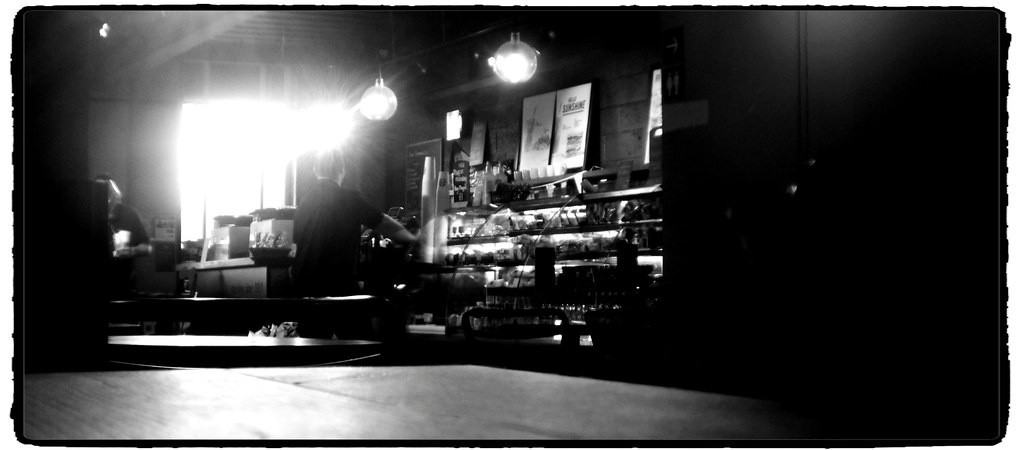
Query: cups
514,164,567,181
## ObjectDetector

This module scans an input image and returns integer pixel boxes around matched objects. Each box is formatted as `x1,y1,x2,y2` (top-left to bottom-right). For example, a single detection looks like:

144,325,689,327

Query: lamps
488,31,537,83
360,67,397,121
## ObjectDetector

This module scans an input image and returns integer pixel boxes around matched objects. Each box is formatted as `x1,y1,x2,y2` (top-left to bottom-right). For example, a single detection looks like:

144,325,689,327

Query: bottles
533,304,621,324
464,302,487,330
482,161,511,205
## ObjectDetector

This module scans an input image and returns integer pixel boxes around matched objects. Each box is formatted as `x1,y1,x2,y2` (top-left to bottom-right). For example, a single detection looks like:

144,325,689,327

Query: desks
22,365,770,445
108,335,382,346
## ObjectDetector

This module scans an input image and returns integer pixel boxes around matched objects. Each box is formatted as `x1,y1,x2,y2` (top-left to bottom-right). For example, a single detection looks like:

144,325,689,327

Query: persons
289,148,421,339
109,180,152,298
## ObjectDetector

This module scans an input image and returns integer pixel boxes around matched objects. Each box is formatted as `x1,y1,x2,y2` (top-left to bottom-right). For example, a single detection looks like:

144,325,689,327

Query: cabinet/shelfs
402,183,663,346
